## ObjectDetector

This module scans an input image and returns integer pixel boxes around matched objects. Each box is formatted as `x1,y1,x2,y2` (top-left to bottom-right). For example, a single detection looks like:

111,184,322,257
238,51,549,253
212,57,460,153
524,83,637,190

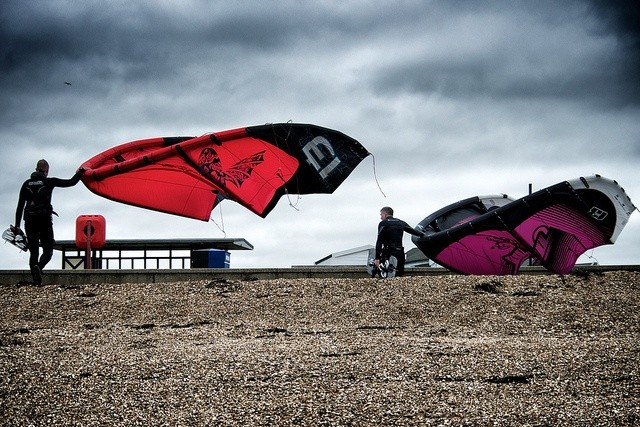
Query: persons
372,207,427,278
14,158,92,283
